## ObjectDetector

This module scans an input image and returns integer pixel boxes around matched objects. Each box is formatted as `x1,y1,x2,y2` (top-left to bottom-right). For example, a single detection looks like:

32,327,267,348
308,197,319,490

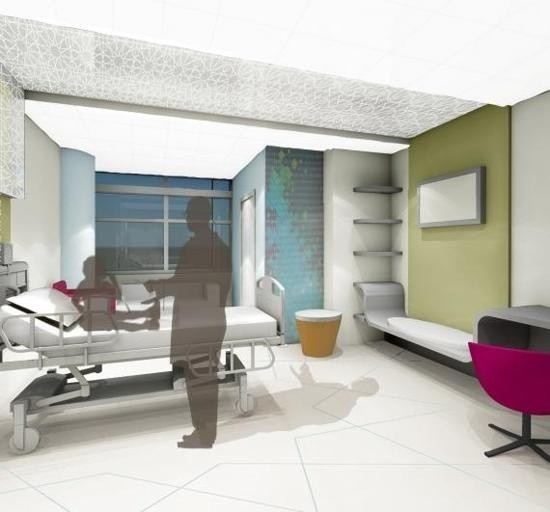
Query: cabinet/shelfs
352,185,404,257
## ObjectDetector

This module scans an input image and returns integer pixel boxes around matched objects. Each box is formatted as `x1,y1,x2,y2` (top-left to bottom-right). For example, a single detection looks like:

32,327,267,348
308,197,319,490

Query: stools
294,308,343,357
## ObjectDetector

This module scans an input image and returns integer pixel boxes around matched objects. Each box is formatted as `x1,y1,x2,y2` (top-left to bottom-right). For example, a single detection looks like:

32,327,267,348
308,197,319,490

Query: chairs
468,341,550,464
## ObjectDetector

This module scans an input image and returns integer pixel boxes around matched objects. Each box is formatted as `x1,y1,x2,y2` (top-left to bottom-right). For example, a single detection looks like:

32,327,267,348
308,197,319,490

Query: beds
0,277,286,456
